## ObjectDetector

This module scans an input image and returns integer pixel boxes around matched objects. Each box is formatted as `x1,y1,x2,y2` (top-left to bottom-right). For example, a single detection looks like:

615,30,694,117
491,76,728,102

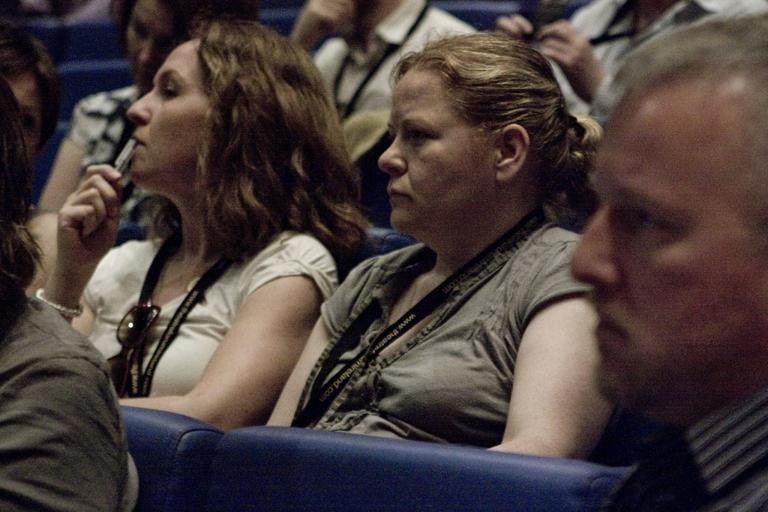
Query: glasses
107,304,161,397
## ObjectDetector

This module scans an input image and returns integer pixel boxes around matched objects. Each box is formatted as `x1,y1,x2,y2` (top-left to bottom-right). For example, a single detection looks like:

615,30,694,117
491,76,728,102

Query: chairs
346,226,415,272
434,3,518,31
255,9,301,36
29,19,134,207
118,404,625,511
